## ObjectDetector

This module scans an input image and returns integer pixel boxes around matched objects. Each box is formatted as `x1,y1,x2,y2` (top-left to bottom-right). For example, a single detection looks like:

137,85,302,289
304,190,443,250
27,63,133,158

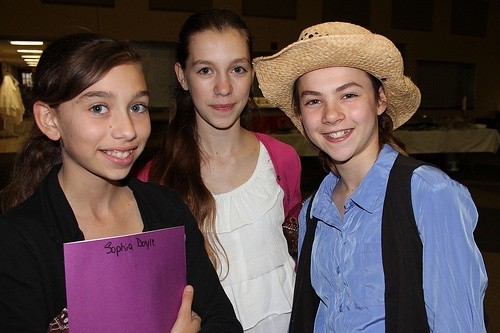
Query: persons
252,19,489,333
1,24,244,333
133,8,302,333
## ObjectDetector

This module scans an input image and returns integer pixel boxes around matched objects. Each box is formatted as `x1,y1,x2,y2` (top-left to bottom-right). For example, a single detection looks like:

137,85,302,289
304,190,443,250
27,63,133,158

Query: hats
252,21,421,136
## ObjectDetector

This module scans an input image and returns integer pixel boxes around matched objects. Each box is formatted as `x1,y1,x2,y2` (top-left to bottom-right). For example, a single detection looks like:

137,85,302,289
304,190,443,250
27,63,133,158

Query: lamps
10,40,44,67
